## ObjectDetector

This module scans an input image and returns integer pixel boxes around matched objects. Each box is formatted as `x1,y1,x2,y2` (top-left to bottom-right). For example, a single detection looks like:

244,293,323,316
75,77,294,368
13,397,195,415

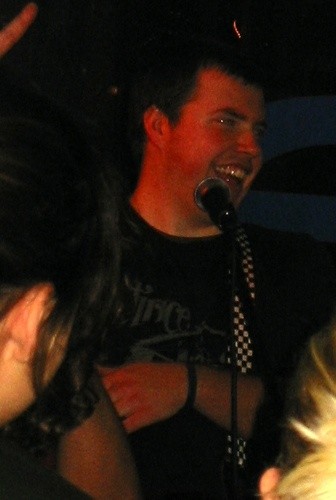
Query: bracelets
180,363,199,409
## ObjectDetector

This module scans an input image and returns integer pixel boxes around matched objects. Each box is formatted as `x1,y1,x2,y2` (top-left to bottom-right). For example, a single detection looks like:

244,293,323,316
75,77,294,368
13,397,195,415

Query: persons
0,4,119,500
45,32,336,500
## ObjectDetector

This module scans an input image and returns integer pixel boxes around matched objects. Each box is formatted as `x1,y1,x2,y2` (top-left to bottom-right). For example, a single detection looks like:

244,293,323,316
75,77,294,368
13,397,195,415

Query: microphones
194,176,237,231
